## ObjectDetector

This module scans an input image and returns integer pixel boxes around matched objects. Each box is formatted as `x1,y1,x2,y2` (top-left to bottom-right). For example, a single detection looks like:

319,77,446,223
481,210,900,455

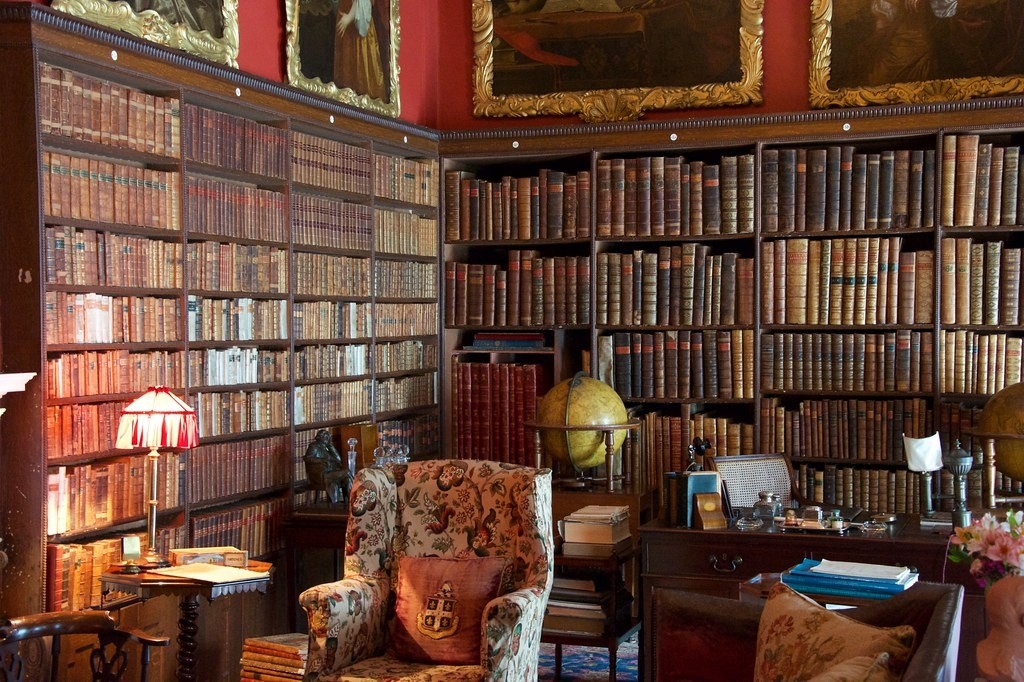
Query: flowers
944,508,1023,593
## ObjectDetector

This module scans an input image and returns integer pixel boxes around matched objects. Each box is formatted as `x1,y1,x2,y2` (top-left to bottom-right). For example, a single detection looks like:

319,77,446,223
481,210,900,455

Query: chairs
0,609,171,682
707,451,822,519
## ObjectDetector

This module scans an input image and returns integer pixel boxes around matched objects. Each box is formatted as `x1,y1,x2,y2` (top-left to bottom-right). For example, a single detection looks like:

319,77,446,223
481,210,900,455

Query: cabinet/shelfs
550,484,653,642
0,0,439,682
443,108,1024,515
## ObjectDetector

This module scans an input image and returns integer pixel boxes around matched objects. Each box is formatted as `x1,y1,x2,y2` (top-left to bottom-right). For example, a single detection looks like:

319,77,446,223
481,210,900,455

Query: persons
305,430,352,506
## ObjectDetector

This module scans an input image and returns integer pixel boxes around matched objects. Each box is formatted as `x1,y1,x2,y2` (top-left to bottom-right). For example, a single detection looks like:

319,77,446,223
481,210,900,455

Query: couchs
648,580,965,682
299,456,554,682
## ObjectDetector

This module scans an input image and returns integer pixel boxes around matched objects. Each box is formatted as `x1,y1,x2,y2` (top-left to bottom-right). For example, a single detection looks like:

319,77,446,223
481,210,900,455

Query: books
939,330,1022,393
626,404,755,522
599,329,753,398
451,332,553,470
558,505,633,557
762,146,935,231
760,397,931,462
793,464,920,515
941,135,1024,227
598,243,753,326
761,237,933,325
778,558,919,601
941,403,1024,512
941,238,1024,326
39,65,439,612
761,330,933,392
542,579,607,636
445,170,590,238
239,633,309,682
444,251,589,326
596,155,756,236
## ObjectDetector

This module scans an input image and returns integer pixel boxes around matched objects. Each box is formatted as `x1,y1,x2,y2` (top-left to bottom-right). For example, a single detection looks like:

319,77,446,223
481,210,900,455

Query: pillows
383,555,508,663
753,580,917,682
797,651,894,681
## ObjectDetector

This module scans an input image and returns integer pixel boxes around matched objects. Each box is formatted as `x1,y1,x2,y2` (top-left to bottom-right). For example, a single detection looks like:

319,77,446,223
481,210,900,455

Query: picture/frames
50,0,240,70
471,0,766,128
808,1,1024,110
282,0,401,119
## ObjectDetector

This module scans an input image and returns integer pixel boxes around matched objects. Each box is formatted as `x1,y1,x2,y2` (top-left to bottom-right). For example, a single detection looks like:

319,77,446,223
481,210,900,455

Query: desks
99,558,278,682
538,539,643,682
637,505,988,682
280,500,348,633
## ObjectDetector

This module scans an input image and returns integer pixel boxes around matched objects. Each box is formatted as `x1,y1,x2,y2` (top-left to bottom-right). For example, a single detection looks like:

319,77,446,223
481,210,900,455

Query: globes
523,371,643,493
960,380,1023,511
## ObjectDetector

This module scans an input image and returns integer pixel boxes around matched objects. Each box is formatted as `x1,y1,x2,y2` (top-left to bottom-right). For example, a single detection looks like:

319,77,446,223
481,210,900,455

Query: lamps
114,384,199,568
